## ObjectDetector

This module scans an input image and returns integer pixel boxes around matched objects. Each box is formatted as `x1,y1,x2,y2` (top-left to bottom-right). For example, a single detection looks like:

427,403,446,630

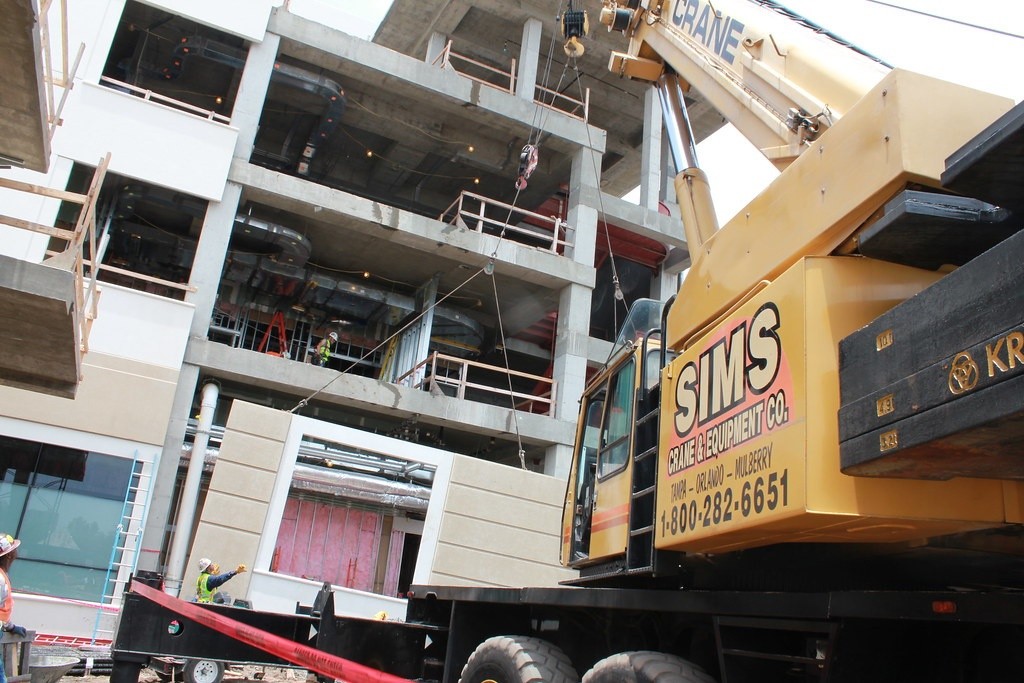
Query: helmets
329,332,338,341
0,532,21,557
197,558,212,573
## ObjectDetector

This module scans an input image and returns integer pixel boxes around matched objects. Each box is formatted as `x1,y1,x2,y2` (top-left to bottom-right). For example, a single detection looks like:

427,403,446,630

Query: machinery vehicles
405,0,1024,683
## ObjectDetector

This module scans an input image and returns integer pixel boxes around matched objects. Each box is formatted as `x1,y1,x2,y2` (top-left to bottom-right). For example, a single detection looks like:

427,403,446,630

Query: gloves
214,564,220,574
8,624,27,638
236,564,248,574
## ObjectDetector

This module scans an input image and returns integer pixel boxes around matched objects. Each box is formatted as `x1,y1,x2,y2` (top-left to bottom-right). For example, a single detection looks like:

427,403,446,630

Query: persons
316,332,338,367
0,532,27,683
196,558,247,603
